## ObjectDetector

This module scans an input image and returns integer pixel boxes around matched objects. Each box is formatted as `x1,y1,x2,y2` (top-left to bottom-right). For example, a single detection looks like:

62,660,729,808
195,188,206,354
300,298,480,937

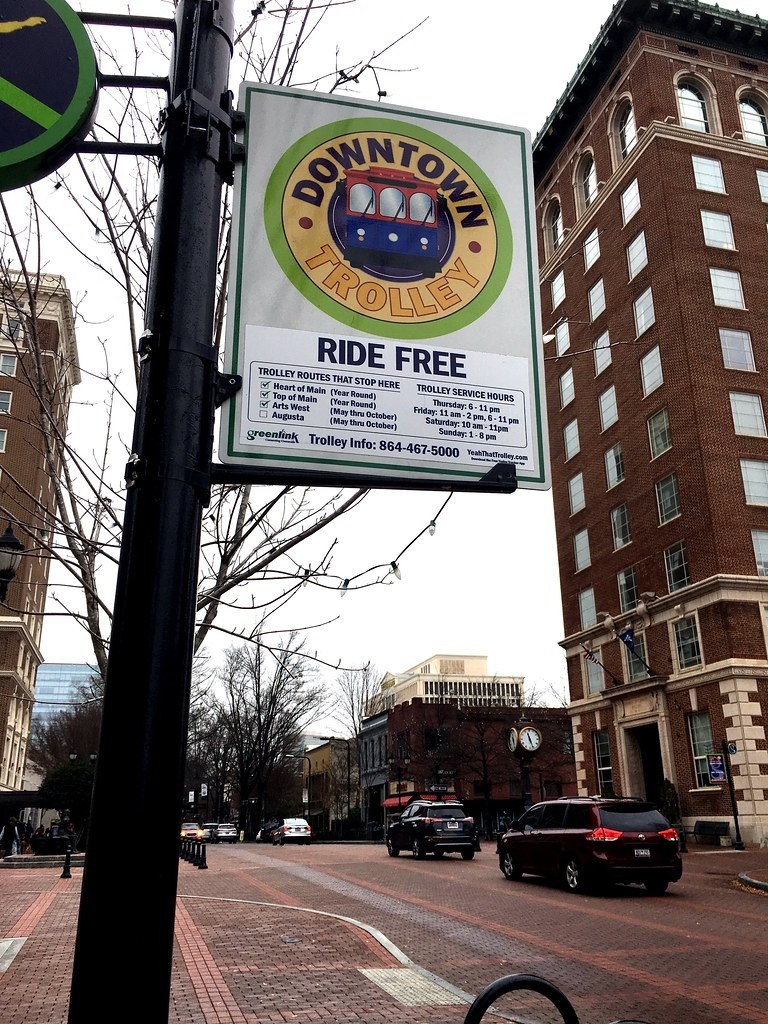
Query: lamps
664,115,676,124
732,131,744,138
640,591,656,602
595,611,610,619
563,227,571,235
597,181,605,189
637,126,646,135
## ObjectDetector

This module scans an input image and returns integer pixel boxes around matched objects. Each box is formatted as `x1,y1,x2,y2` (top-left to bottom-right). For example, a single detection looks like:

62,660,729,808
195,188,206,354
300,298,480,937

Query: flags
619,629,634,654
583,653,599,664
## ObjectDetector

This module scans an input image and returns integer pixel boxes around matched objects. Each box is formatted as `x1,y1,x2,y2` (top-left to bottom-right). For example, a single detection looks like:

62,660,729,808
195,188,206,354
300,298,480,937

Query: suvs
386,800,480,861
495,795,682,894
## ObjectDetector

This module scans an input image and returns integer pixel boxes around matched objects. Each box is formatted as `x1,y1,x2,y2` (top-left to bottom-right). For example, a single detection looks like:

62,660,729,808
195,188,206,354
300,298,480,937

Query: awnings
293,807,327,817
442,794,457,801
420,795,437,800
381,796,414,807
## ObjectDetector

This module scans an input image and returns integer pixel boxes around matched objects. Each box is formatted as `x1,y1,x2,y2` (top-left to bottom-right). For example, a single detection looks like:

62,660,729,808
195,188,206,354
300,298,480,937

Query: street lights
313,735,350,840
286,754,311,820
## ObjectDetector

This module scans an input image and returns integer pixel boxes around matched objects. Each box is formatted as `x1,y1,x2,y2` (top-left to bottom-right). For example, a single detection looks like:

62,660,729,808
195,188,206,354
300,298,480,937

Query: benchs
686,821,729,845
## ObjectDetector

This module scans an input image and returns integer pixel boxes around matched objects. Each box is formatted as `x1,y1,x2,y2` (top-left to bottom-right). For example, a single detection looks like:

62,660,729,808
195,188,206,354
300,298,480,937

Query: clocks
507,728,518,753
518,727,541,751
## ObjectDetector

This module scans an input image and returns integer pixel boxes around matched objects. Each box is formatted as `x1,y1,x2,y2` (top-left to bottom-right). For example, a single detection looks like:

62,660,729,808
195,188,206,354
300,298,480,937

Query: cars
201,823,219,842
211,823,239,844
270,818,312,845
180,823,202,842
256,819,278,843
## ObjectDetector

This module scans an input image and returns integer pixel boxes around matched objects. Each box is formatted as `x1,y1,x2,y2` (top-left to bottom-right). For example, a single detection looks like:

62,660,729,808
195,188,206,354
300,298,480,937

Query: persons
17,821,50,853
0,817,21,859
659,779,688,853
